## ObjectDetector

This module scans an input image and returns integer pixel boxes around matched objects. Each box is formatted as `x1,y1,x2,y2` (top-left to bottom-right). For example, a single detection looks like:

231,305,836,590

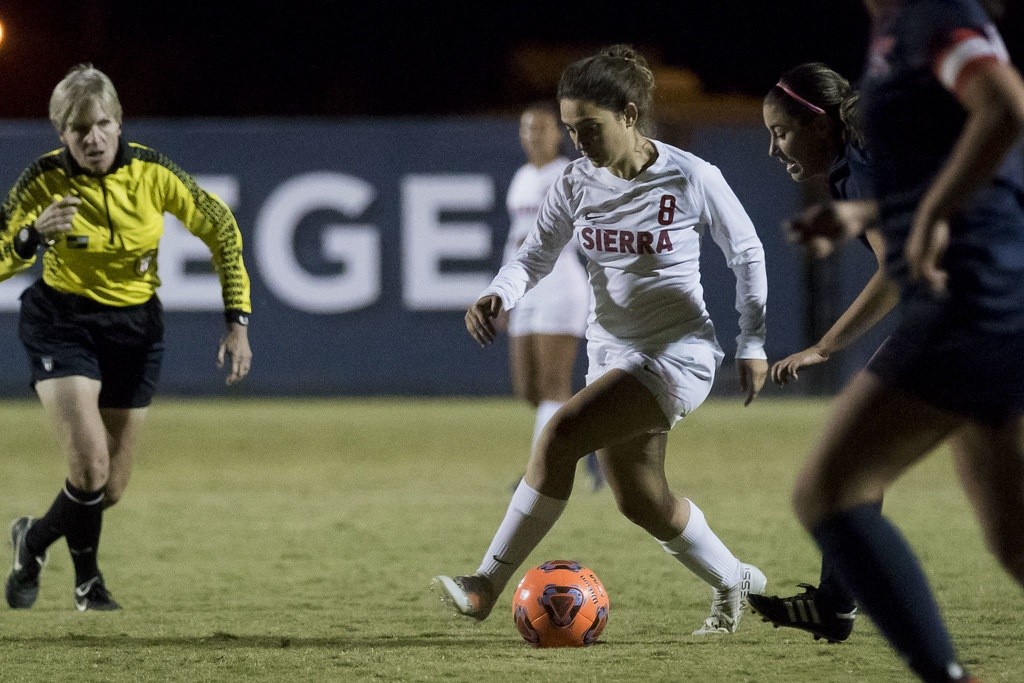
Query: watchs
223,312,250,325
19,226,37,250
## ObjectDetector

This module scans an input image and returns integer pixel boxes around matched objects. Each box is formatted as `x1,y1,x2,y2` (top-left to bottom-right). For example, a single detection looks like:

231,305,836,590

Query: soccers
510,557,610,649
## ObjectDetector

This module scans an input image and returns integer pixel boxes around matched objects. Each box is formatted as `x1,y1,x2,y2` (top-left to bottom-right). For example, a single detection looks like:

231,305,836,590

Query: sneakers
74,577,122,611
4,516,46,610
430,574,497,623
692,561,767,637
747,583,858,643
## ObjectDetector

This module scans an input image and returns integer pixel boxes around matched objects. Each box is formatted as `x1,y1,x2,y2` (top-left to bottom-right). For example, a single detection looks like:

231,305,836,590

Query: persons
428,47,767,639
797,1,1024,683
502,103,603,499
0,65,251,613
743,60,992,643
